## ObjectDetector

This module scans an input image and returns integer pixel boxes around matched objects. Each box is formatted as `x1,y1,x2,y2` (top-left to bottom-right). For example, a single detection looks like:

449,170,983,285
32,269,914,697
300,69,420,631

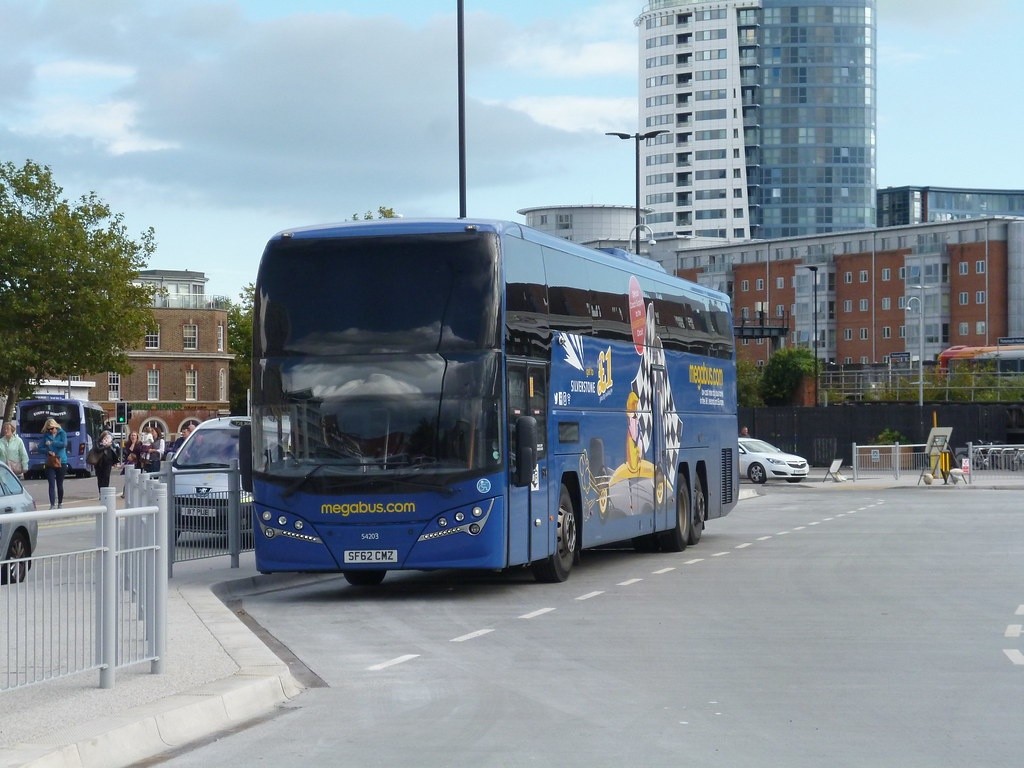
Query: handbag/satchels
87,447,105,465
7,459,22,474
46,455,62,468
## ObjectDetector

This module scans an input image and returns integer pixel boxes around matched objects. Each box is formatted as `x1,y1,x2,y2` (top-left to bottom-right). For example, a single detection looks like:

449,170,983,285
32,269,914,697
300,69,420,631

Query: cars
737,438,810,484
170,415,290,541
0,462,39,584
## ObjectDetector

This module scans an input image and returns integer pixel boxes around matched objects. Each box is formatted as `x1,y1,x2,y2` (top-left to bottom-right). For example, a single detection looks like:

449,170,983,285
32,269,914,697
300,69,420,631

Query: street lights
907,296,923,406
604,129,670,255
806,265,819,406
629,223,657,253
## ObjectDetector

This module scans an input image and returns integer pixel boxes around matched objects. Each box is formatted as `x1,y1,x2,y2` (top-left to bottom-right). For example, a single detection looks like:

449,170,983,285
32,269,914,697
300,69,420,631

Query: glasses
47,427,54,430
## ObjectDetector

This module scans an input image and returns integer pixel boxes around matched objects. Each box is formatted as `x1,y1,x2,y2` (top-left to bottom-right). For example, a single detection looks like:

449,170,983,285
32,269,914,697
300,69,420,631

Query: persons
0,422,29,479
140,426,165,479
121,431,142,499
94,429,120,493
37,419,68,510
173,426,190,452
738,426,750,438
188,425,203,445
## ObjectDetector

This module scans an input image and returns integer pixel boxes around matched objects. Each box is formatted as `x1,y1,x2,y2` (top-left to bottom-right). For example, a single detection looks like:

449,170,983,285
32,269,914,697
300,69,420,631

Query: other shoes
49,505,56,510
121,495,125,498
58,503,62,509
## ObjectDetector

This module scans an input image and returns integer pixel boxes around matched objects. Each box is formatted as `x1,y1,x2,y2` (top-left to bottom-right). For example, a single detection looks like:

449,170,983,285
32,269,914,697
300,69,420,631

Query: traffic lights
127,402,133,424
115,402,127,424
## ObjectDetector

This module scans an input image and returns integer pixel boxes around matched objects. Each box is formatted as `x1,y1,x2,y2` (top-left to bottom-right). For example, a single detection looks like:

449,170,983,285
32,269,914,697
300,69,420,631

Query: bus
14,397,112,480
251,218,739,587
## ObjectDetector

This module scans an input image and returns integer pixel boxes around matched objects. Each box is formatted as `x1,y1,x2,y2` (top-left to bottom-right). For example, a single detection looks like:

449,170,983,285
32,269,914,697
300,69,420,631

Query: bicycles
953,440,1024,471
